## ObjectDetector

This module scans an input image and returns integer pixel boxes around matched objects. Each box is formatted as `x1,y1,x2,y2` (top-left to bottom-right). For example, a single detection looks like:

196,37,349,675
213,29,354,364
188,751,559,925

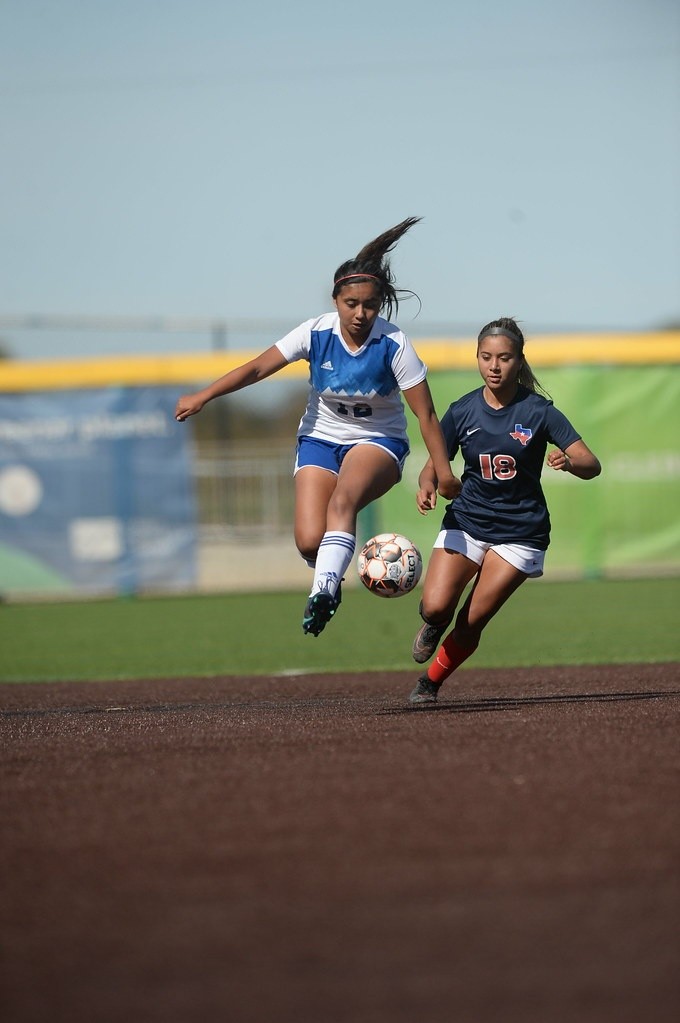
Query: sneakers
413,613,456,663
333,578,344,612
303,591,334,637
409,669,444,703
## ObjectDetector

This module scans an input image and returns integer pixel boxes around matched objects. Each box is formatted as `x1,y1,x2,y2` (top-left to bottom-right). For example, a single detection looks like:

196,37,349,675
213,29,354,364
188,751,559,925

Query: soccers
357,533,424,599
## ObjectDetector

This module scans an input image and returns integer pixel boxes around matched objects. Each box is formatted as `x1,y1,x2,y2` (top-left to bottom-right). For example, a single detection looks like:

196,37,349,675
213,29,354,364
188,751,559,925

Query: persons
175,217,464,638
409,317,602,704
